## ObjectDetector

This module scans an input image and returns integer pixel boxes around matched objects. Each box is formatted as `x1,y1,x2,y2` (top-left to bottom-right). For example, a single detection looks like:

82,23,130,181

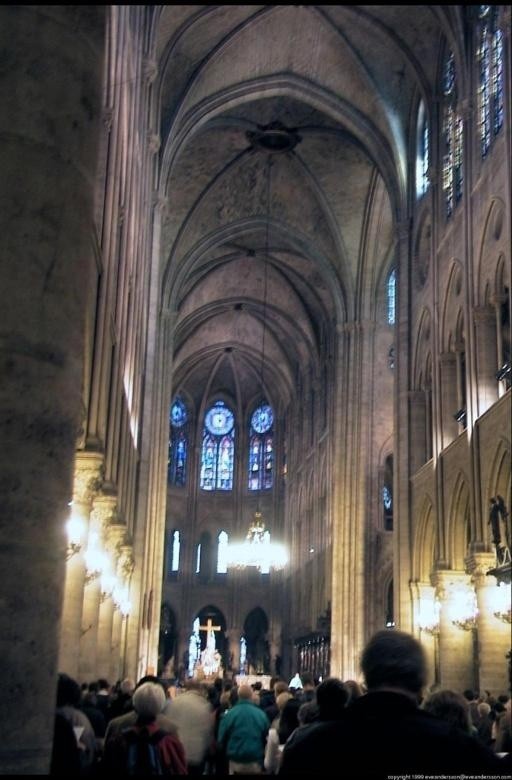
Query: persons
51,631,512,780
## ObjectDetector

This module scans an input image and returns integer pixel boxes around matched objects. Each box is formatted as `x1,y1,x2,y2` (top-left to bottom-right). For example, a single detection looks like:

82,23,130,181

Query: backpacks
122,728,169,780
